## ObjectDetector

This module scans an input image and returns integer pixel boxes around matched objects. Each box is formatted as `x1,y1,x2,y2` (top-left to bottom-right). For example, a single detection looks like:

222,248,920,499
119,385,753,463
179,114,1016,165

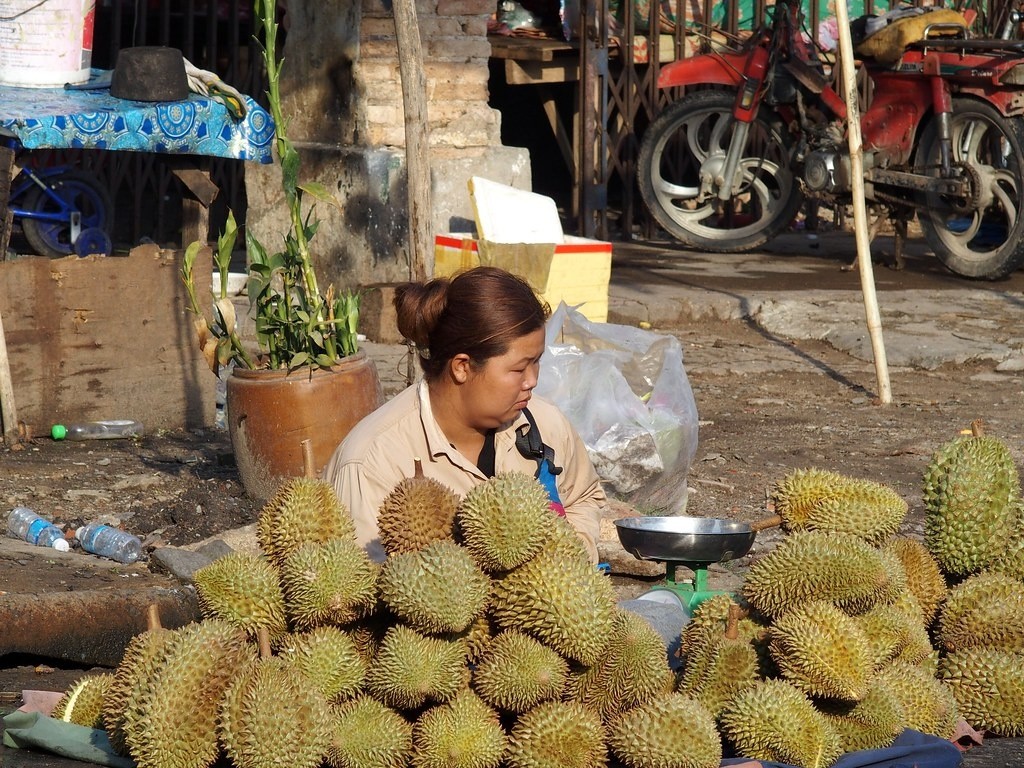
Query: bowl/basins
109,47,188,101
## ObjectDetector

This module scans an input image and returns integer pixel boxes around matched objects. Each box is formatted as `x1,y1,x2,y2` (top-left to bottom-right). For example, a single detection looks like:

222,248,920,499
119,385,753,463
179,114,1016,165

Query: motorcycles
635,0,1024,282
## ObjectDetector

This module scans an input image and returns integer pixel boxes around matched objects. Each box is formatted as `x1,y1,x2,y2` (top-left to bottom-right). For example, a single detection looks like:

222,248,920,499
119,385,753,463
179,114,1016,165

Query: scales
615,516,785,669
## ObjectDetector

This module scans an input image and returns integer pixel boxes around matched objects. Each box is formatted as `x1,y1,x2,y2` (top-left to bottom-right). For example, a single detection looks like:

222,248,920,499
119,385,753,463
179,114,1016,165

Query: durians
48,422,1024,768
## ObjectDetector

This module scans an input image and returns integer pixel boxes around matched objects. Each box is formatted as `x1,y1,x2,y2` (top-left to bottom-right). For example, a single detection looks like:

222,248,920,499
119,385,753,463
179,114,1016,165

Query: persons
326,267,606,568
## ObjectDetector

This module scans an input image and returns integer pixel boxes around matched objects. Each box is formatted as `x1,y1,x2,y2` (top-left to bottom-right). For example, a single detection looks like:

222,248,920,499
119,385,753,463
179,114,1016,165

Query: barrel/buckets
0,0,95,88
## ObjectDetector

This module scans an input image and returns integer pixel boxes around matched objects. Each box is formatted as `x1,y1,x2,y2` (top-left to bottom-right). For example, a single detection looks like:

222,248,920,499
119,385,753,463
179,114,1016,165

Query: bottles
75,522,142,564
52,418,143,441
6,507,69,553
